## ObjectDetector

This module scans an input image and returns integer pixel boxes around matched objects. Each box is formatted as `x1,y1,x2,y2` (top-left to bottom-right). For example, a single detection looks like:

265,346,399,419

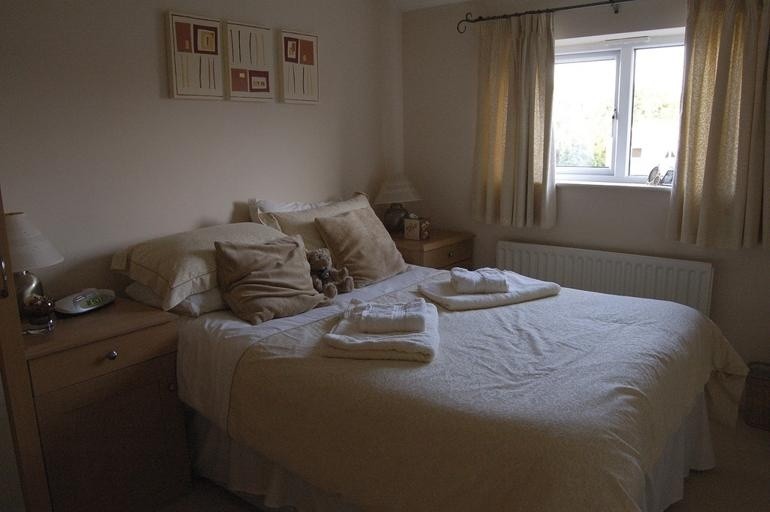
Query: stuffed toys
305,247,354,299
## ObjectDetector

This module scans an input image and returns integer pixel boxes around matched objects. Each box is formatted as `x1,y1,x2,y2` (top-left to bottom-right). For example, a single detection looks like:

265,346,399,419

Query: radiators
495,238,715,318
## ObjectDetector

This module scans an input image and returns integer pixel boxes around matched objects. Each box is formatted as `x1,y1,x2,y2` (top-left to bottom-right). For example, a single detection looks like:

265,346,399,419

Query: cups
22,297,57,335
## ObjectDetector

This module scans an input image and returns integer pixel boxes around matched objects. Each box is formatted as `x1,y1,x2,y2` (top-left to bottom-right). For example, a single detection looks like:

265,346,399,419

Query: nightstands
394,228,476,270
23,295,193,512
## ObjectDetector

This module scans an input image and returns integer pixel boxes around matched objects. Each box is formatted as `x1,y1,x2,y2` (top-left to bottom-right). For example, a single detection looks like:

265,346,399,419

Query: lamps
372,168,423,240
5,212,64,321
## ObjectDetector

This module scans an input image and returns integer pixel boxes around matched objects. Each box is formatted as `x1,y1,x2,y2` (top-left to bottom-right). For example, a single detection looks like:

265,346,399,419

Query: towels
321,296,440,363
417,270,563,311
358,297,426,334
449,266,510,295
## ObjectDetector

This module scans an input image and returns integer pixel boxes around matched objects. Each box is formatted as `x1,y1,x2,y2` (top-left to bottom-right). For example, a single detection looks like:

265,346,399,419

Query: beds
109,262,752,512
